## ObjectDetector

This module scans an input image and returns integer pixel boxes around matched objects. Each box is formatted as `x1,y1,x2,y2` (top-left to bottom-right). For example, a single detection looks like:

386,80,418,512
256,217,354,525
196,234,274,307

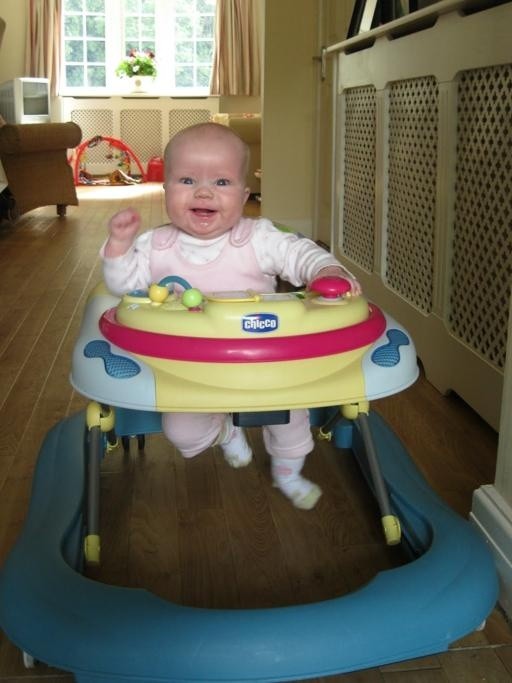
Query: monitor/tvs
0,78,51,123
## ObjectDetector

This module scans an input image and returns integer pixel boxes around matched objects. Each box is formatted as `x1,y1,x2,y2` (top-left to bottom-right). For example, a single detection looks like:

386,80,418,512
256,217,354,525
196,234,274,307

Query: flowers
116,49,157,79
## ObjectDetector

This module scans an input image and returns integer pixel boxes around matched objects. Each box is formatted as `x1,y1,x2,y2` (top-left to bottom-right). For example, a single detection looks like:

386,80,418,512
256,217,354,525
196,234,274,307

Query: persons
100,124,363,515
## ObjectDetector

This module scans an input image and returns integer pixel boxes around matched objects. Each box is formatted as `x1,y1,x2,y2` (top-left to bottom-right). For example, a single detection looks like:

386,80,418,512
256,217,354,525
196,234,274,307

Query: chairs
0,121,82,219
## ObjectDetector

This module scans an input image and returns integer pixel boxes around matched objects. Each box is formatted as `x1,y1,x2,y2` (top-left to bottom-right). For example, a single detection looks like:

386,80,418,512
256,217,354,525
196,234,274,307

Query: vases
130,77,149,93
